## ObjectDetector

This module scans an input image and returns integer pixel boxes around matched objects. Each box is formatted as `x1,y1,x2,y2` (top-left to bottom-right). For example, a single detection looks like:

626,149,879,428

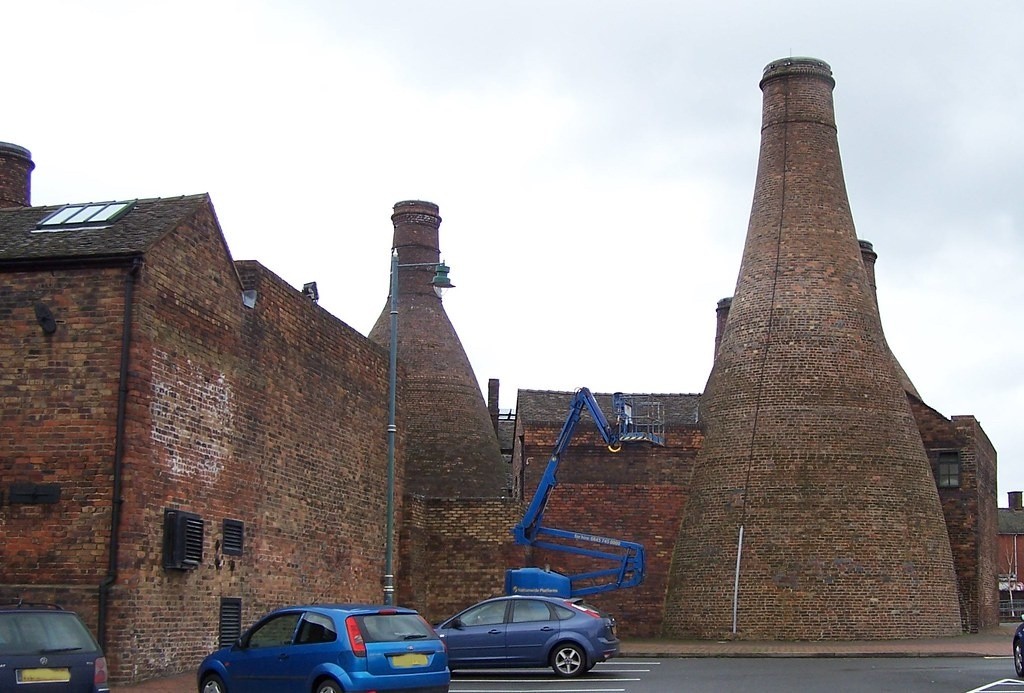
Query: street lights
384,249,455,607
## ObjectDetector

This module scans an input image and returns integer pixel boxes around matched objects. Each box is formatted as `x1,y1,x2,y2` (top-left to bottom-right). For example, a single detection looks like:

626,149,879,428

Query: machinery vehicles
505,385,668,604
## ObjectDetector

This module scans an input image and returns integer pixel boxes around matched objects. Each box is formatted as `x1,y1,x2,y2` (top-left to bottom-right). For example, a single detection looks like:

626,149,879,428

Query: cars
1012,613,1024,677
196,603,450,693
434,596,622,678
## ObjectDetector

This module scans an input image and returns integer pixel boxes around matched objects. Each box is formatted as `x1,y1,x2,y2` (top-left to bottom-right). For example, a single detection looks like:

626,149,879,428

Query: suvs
0,599,110,693
